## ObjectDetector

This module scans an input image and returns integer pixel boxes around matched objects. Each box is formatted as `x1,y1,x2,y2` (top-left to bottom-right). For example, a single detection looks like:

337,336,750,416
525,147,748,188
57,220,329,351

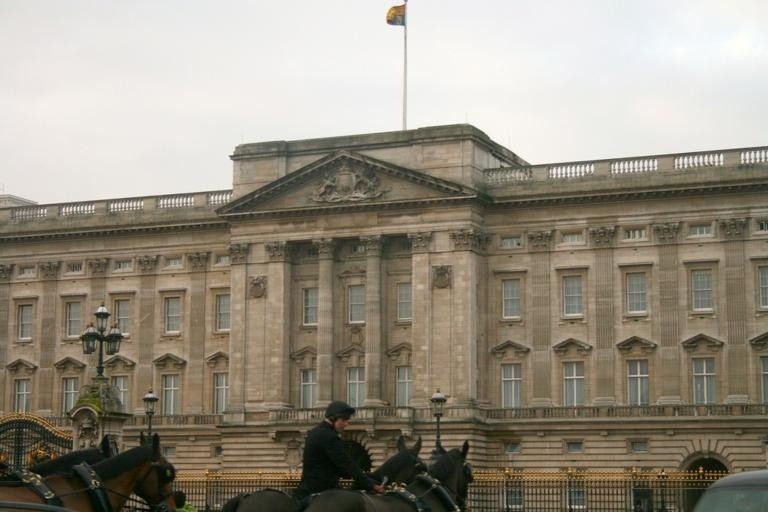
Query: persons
292,400,389,502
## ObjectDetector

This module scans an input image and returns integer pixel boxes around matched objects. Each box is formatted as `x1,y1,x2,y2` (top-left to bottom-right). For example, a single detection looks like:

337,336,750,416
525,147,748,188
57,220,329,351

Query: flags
386,3,406,27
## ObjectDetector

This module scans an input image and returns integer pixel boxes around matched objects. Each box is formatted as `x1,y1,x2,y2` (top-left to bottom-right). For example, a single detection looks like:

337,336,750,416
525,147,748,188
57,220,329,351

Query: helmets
325,401,355,421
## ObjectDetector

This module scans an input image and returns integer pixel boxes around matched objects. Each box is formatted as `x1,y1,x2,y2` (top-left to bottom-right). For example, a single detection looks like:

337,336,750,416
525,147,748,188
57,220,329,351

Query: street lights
427,386,448,461
142,386,159,443
79,302,128,394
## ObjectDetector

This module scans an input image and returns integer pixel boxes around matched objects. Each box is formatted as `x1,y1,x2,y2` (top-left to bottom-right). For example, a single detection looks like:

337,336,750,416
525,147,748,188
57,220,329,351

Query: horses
221,435,423,512
0,429,178,512
303,435,472,512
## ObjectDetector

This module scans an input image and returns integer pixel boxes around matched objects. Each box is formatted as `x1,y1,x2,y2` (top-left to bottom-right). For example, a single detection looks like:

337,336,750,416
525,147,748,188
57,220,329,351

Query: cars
687,467,768,512
